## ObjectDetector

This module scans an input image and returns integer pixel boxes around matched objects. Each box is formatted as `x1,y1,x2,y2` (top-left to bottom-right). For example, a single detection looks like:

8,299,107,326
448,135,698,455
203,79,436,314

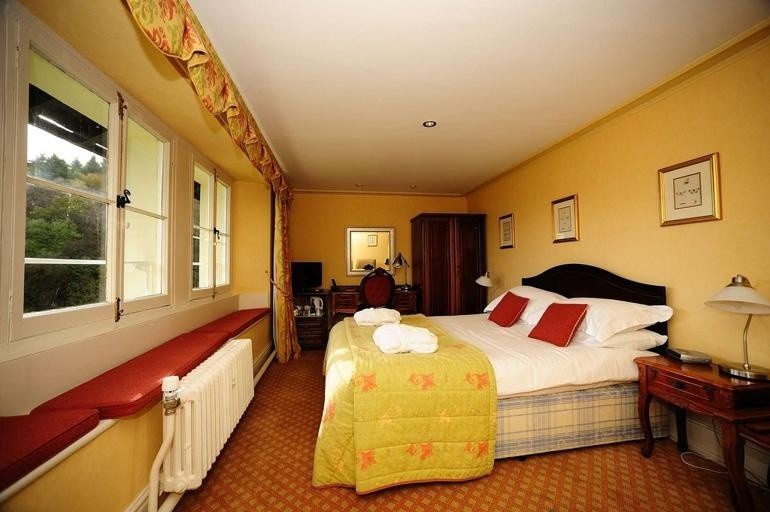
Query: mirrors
346,227,395,277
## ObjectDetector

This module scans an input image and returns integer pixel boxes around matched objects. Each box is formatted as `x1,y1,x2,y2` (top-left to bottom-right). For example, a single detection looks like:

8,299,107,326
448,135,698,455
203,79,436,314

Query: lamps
392,252,411,290
704,275,770,381
476,272,500,302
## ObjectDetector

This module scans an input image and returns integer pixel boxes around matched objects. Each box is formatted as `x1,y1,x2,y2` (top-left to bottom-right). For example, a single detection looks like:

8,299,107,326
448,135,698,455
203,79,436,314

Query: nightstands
633,356,770,512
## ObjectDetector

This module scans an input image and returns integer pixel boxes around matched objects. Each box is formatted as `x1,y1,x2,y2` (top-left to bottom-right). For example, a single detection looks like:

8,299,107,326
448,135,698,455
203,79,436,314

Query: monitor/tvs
291,261,322,292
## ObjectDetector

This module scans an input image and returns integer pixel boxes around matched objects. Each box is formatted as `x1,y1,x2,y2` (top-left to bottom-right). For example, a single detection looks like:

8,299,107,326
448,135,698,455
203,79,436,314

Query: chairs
356,267,395,312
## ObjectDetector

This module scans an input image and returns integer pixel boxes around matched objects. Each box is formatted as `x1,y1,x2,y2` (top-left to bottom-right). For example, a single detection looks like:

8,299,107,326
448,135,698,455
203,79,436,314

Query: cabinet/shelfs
357,259,376,269
292,290,330,347
410,213,487,316
330,285,416,327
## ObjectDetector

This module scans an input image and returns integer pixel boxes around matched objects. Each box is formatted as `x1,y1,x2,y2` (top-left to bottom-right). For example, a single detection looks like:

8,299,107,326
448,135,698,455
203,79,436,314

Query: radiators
148,339,255,511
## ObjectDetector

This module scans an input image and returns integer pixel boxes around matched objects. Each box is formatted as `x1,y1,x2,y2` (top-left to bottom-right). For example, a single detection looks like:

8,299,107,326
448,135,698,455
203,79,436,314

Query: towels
372,324,439,354
354,307,401,326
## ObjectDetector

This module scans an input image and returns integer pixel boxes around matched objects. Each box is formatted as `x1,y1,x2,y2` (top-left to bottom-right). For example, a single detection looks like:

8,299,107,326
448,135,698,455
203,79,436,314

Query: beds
311,265,670,494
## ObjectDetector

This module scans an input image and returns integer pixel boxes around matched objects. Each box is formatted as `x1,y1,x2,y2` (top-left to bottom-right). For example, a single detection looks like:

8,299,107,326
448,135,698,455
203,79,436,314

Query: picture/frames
658,152,722,227
368,235,378,247
499,213,515,249
551,193,580,243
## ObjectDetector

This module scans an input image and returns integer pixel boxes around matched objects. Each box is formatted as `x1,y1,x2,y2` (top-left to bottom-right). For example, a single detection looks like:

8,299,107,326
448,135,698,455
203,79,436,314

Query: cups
296,305,324,316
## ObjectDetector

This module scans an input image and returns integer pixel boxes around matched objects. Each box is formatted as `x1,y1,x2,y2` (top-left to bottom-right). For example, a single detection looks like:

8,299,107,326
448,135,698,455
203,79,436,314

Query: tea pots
311,295,323,316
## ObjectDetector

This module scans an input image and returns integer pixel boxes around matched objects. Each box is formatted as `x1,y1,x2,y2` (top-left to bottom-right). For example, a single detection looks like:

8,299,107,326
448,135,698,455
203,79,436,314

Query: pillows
484,286,674,350
